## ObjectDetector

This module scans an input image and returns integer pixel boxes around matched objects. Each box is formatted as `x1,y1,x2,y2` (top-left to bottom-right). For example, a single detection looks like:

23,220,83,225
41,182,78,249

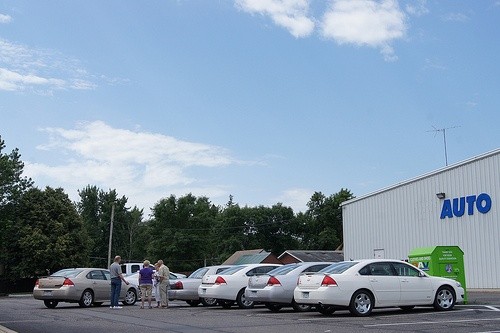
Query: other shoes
141,306,144,309
113,305,123,309
149,306,152,309
154,304,168,309
110,306,113,309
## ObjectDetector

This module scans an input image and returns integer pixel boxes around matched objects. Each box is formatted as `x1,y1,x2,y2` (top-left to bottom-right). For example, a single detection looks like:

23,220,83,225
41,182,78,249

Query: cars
293,259,465,317
33,267,139,310
168,266,237,306
244,261,341,313
125,271,186,302
197,263,285,309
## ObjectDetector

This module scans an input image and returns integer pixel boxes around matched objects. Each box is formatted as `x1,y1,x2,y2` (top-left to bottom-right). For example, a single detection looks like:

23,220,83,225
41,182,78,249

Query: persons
109,255,130,309
139,260,170,309
